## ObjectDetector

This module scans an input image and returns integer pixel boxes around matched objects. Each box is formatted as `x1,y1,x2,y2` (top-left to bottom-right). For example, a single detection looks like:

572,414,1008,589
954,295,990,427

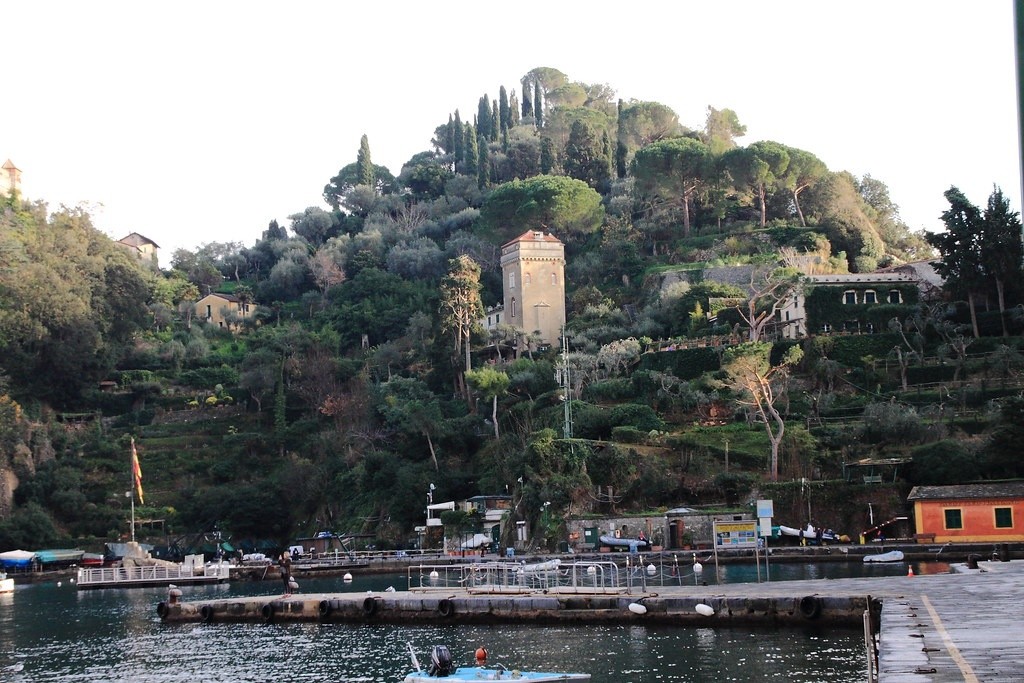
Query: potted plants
681,531,692,550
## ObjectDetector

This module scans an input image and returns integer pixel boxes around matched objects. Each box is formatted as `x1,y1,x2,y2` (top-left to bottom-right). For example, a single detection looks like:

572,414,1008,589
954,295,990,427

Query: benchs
913,532,935,542
576,541,595,552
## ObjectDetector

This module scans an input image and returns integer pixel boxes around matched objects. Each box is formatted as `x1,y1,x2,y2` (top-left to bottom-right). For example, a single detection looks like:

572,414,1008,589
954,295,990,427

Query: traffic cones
906,565,914,578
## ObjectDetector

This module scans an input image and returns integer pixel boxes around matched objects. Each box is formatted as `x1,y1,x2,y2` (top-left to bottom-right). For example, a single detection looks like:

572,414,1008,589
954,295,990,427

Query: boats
779,526,851,541
402,642,591,683
523,559,562,571
863,550,904,562
599,535,653,546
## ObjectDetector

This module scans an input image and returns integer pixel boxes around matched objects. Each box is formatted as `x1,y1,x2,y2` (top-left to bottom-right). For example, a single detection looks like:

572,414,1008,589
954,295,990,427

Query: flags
131,440,144,503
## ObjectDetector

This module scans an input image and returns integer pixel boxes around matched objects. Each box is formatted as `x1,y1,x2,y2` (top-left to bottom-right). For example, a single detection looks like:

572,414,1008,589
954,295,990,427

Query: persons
799,527,805,546
283,549,291,563
816,527,826,547
481,545,486,557
293,548,299,560
878,524,884,546
429,646,452,678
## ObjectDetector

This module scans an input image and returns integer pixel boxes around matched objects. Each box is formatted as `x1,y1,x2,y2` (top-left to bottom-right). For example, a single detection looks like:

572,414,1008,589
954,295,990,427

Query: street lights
540,500,551,525
